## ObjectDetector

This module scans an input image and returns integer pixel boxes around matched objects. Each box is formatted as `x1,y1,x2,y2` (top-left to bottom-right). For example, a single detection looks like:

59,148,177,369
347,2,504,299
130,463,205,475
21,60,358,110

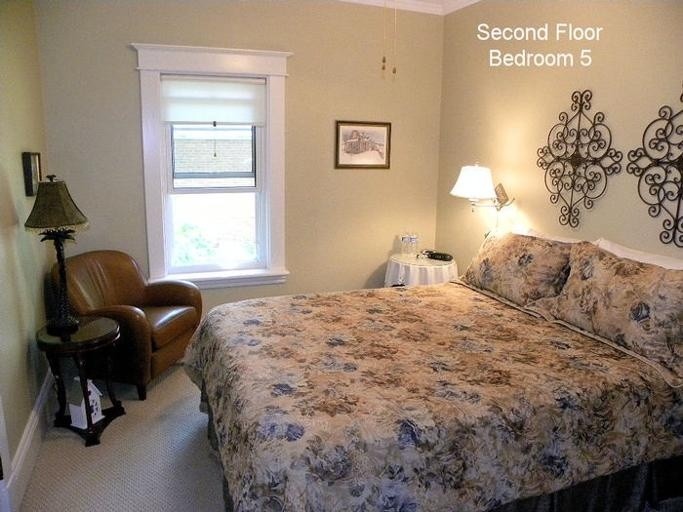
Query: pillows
453,226,683,389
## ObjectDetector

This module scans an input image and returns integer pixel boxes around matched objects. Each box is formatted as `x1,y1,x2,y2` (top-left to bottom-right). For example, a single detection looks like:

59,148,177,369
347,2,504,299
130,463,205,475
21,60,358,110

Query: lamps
24,173,91,337
449,161,509,213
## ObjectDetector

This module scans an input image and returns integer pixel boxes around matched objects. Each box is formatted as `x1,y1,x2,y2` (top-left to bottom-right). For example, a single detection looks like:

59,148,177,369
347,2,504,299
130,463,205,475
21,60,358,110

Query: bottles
399,233,416,261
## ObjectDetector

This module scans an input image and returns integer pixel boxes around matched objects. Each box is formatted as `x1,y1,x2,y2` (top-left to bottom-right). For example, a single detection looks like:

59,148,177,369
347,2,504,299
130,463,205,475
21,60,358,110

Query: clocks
67,378,105,430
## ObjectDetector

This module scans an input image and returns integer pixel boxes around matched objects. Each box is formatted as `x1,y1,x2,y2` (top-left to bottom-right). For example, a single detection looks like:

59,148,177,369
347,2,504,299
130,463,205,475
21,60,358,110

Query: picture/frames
22,152,42,196
335,120,391,169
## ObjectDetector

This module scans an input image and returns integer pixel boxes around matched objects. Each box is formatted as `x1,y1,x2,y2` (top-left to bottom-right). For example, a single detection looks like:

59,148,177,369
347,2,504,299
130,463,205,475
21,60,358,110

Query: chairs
49,250,202,402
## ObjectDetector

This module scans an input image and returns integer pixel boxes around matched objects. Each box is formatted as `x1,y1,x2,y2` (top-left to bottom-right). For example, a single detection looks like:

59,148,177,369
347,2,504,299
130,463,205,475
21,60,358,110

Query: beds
176,281,683,512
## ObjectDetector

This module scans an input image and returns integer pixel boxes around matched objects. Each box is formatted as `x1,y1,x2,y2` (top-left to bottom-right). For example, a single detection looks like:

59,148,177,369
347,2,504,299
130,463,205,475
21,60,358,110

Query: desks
384,254,458,287
35,316,127,448
